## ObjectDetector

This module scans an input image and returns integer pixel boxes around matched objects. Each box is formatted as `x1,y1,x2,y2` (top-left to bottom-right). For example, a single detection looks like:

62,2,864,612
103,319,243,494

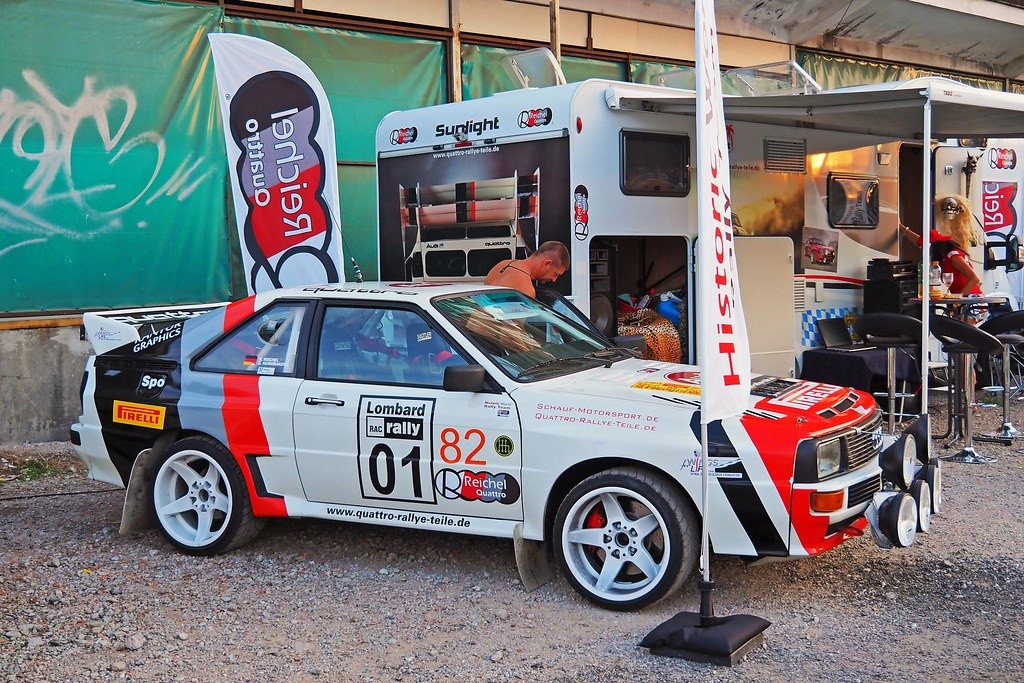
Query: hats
918,229,952,246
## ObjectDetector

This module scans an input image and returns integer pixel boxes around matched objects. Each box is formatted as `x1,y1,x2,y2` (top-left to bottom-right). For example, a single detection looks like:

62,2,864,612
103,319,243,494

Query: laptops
817,318,877,352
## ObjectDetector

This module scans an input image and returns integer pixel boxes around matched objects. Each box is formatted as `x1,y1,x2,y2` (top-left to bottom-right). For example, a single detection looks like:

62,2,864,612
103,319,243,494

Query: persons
895,217,990,414
463,241,570,378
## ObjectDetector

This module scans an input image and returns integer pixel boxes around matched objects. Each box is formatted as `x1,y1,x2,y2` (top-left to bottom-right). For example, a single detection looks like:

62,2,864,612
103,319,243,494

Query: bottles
918,261,923,299
930,261,942,300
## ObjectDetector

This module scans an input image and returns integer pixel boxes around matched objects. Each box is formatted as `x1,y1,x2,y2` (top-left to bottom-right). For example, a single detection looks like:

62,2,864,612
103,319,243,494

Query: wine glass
942,273,953,293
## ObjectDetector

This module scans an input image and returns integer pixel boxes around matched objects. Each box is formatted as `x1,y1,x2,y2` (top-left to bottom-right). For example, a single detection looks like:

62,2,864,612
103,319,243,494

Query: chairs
318,327,393,383
403,321,463,387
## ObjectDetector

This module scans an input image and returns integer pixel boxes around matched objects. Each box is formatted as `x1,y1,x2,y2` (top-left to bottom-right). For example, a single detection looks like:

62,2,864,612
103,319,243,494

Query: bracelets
904,227,909,236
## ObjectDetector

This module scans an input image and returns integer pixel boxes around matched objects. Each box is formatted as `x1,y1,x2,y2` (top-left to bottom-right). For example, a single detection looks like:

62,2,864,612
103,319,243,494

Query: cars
69,281,942,613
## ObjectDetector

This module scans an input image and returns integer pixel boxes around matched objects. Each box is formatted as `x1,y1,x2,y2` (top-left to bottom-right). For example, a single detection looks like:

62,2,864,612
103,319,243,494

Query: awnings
623,75,1023,458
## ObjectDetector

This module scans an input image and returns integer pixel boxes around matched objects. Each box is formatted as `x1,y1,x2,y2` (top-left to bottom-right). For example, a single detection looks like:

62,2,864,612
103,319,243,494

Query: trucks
374,48,1024,378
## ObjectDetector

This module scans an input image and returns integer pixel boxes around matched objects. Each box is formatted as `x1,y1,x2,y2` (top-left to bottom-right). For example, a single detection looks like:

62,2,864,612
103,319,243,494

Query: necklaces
500,259,532,275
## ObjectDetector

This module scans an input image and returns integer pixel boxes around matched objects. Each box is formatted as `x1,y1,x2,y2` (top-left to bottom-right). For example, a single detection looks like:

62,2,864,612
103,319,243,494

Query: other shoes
961,405,977,414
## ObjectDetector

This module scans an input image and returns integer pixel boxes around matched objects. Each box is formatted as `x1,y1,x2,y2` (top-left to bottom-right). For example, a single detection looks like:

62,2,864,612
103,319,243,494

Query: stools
854,310,1024,464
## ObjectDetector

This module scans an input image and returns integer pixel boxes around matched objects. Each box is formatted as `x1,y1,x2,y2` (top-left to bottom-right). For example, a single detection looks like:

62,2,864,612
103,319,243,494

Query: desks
800,346,921,424
909,297,1011,450
